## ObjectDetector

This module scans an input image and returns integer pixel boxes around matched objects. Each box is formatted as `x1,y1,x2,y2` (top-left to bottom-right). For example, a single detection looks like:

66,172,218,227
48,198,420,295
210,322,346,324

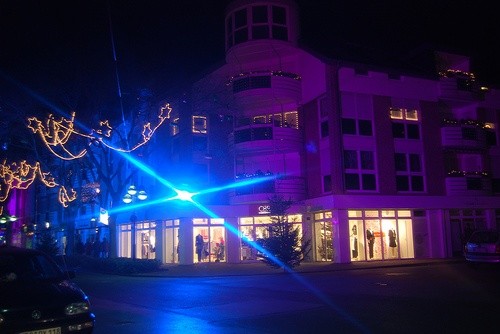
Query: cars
0,244,96,334
463,230,500,269
